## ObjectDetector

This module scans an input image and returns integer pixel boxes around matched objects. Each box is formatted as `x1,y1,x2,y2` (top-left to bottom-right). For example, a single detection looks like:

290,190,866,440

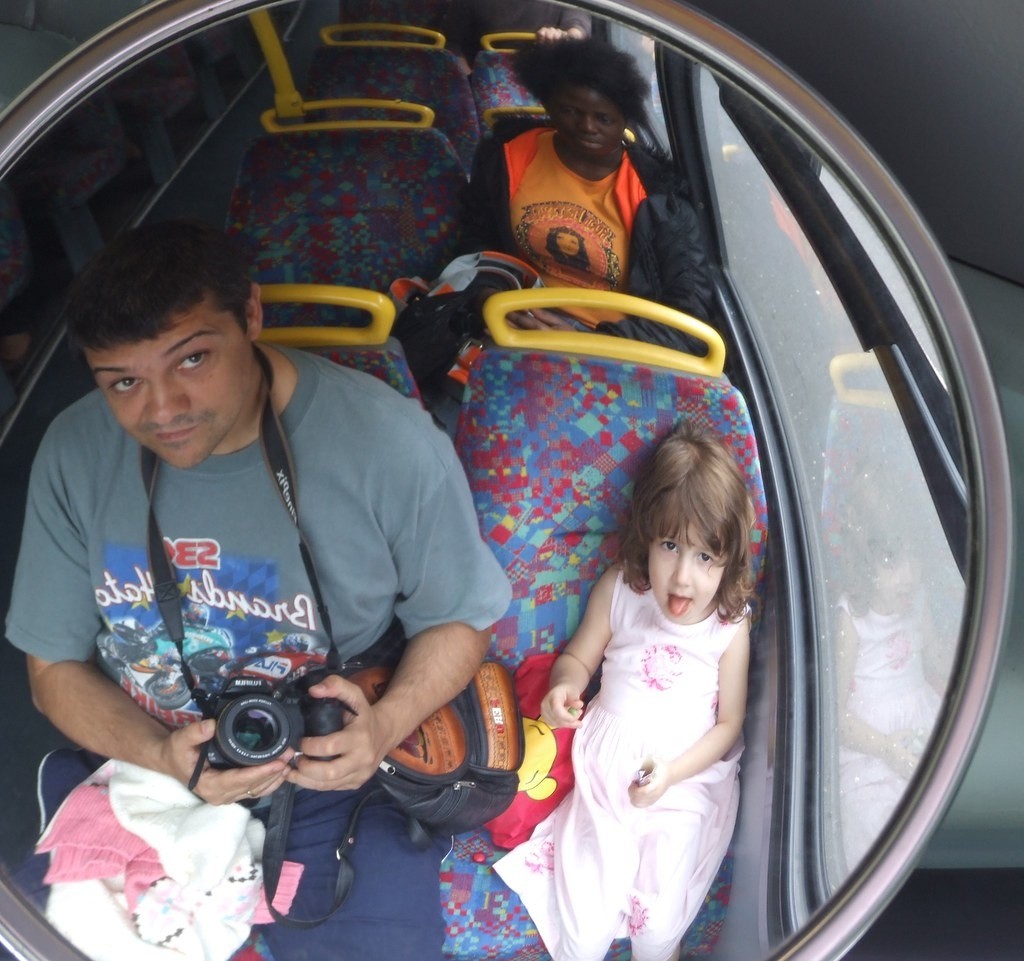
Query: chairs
207,18,769,961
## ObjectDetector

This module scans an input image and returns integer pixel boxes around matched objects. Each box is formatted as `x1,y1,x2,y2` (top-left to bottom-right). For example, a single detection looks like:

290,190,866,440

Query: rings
246,790,257,797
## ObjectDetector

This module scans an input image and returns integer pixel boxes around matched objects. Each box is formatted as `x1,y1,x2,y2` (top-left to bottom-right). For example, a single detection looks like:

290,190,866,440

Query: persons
825,492,945,874
491,421,758,961
4,218,515,961
437,0,718,358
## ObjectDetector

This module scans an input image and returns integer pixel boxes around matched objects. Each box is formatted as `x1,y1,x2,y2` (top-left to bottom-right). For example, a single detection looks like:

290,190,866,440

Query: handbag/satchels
293,659,525,838
388,252,542,402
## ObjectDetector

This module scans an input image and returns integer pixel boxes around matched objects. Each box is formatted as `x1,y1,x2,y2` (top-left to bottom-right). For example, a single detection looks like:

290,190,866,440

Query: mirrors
0,0,989,961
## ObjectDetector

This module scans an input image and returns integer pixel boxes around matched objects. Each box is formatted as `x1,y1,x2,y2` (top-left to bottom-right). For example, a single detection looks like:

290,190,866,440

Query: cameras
205,674,344,771
